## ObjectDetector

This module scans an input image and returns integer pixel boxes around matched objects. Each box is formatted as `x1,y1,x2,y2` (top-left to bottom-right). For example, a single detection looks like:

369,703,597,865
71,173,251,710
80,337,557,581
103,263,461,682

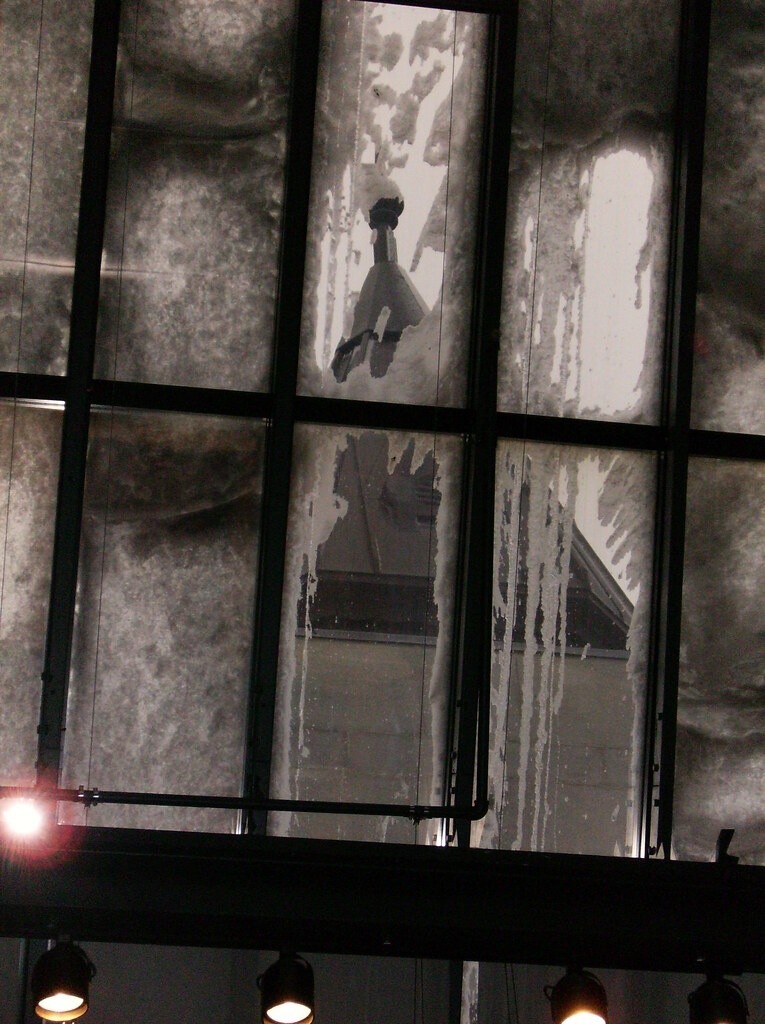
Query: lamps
30,939,97,1023
255,949,315,1024
544,965,609,1024
688,973,750,1024
